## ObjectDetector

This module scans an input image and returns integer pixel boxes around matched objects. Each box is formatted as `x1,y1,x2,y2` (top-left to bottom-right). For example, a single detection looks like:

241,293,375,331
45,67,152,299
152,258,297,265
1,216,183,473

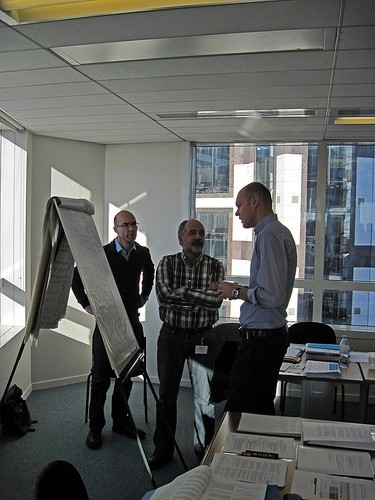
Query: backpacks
0,384,32,436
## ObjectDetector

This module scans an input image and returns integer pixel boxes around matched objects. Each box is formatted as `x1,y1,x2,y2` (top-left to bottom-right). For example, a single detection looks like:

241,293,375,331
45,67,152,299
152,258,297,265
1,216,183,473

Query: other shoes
144,454,173,471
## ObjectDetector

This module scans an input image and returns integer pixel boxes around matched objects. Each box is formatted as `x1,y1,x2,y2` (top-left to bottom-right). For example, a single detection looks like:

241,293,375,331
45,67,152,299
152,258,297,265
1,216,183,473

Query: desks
277,350,375,420
204,407,375,500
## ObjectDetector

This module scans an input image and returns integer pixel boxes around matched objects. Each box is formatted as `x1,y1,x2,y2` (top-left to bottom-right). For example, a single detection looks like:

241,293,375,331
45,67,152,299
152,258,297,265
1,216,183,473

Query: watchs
231,286,242,299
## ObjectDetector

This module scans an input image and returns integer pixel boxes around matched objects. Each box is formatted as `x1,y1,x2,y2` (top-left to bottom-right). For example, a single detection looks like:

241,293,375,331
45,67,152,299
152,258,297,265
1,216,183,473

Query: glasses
116,223,139,228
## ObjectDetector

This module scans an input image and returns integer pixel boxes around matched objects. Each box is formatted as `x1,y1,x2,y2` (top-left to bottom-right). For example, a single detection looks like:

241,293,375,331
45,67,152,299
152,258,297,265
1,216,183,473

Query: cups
368,352,375,369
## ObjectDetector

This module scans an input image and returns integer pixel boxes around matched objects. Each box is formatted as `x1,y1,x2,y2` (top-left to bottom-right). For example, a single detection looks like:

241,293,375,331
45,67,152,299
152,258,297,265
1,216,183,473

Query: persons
72,209,155,450
147,218,225,469
217,182,297,416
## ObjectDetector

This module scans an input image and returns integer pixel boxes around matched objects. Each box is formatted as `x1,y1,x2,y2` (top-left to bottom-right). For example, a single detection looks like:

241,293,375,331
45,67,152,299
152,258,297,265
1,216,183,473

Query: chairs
85,337,147,422
216,323,240,340
28,460,90,500
282,321,345,421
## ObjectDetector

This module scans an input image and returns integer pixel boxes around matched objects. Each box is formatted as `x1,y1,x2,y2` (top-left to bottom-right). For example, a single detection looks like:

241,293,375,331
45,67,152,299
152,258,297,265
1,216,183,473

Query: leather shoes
86,430,102,449
112,424,146,439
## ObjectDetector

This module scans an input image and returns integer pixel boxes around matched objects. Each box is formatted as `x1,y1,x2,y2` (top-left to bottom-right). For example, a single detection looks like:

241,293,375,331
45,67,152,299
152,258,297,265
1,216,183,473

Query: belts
237,325,288,338
163,322,213,335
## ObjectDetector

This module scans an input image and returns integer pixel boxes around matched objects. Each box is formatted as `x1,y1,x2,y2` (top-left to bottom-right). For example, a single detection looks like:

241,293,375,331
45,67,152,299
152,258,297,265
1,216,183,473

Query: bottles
339,335,350,368
